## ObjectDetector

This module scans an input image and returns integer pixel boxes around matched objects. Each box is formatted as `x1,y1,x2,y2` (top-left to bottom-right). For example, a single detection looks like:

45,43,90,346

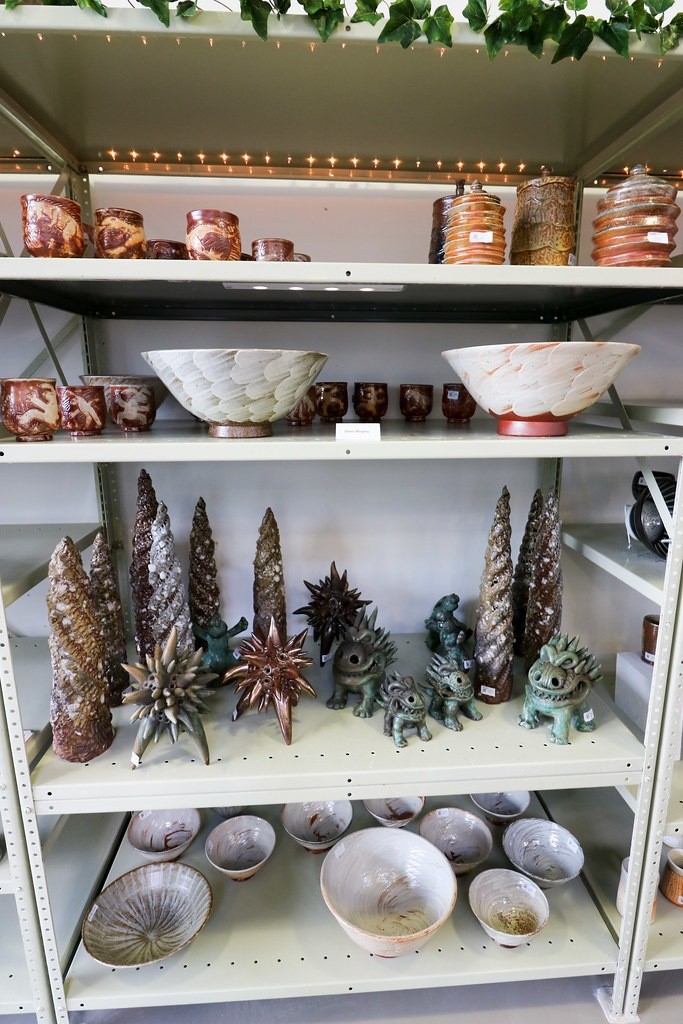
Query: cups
93,208,146,259
146,239,187,260
20,193,85,258
80,221,93,257
316,381,348,423
186,210,252,261
399,384,434,422
109,384,156,432
659,849,683,906
352,382,388,422
56,386,108,436
441,384,477,425
0,378,61,442
284,385,316,427
616,857,629,915
642,615,660,665
252,238,295,262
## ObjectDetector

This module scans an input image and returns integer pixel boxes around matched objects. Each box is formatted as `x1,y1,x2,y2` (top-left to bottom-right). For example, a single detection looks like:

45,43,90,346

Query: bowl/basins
204,815,276,882
78,374,170,431
212,806,247,818
468,869,550,946
281,801,353,854
141,349,330,438
320,826,458,958
420,807,493,872
363,796,426,827
440,342,642,436
469,792,530,821
502,818,584,887
125,808,202,863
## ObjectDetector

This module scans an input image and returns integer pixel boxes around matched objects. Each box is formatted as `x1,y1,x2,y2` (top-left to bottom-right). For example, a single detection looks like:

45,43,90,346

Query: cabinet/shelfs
0,0,683,1024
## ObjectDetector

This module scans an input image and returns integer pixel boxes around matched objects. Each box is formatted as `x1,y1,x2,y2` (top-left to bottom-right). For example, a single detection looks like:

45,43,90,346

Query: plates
81,863,213,968
